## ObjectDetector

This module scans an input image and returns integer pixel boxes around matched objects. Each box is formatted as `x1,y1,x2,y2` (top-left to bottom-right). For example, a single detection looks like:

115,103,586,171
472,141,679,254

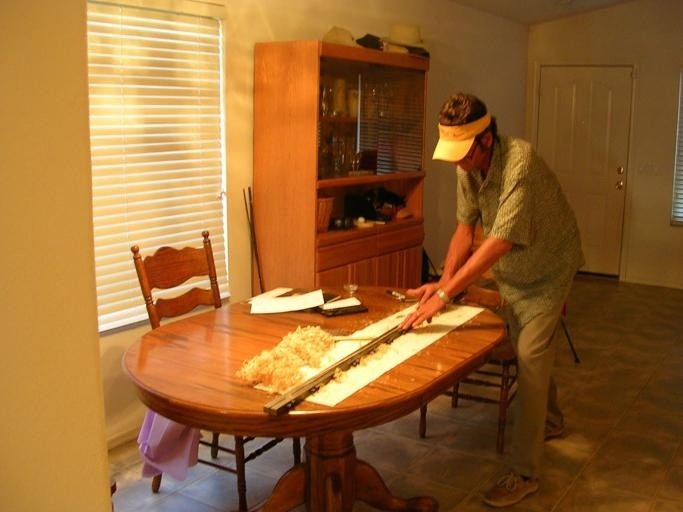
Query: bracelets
436,288,450,304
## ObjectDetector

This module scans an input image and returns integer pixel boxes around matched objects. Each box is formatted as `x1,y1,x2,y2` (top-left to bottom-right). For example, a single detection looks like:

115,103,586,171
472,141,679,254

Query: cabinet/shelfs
254,42,430,296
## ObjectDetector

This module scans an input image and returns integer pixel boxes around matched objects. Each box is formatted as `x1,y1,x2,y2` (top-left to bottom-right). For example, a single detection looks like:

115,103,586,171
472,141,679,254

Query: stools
418,282,521,451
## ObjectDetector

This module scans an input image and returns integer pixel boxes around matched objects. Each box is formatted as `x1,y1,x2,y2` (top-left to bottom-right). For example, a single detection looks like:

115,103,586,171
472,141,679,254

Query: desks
135,283,506,508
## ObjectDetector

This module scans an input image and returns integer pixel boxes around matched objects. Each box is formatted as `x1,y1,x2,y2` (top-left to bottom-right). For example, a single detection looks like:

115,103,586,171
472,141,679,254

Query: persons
399,92,585,508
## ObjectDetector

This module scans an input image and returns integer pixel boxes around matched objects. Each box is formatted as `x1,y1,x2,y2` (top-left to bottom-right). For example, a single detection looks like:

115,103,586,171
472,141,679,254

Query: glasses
464,134,484,160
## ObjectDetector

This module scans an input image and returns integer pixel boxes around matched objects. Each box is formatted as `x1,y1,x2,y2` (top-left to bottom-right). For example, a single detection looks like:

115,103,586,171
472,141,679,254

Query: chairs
128,232,302,510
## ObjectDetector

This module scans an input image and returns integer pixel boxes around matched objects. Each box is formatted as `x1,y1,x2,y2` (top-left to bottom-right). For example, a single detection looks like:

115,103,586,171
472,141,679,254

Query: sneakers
483,470,538,507
544,409,565,436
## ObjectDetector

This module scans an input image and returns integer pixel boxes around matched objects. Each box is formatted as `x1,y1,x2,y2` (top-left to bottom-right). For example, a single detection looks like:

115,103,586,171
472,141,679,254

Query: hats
382,19,425,49
432,111,491,163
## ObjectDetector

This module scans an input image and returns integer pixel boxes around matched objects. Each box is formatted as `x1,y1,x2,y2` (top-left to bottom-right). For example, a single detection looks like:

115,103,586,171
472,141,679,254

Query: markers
386,289,406,299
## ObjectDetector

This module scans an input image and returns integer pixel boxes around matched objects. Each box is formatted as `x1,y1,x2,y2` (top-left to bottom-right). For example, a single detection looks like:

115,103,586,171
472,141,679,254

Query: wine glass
342,263,359,298
318,65,404,178
